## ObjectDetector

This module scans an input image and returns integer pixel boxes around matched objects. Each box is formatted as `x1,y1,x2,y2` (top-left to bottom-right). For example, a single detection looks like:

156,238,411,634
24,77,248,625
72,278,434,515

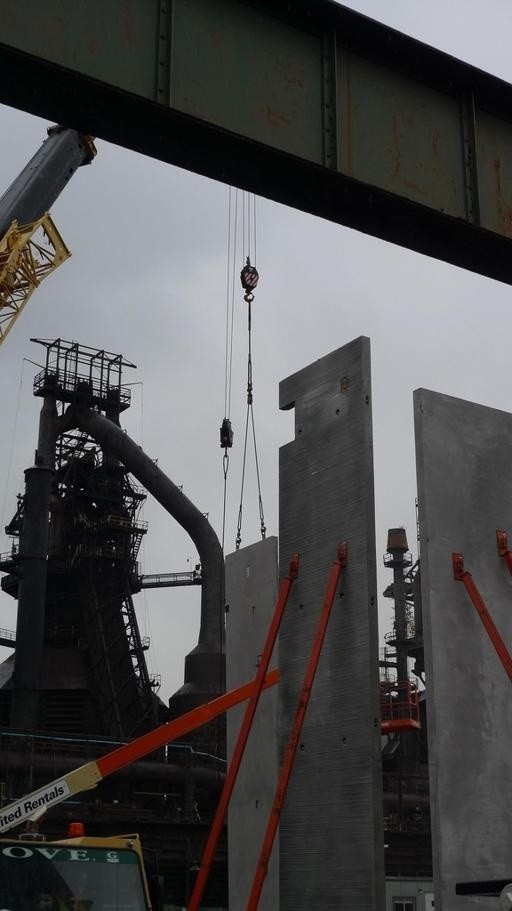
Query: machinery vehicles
0,663,284,911
